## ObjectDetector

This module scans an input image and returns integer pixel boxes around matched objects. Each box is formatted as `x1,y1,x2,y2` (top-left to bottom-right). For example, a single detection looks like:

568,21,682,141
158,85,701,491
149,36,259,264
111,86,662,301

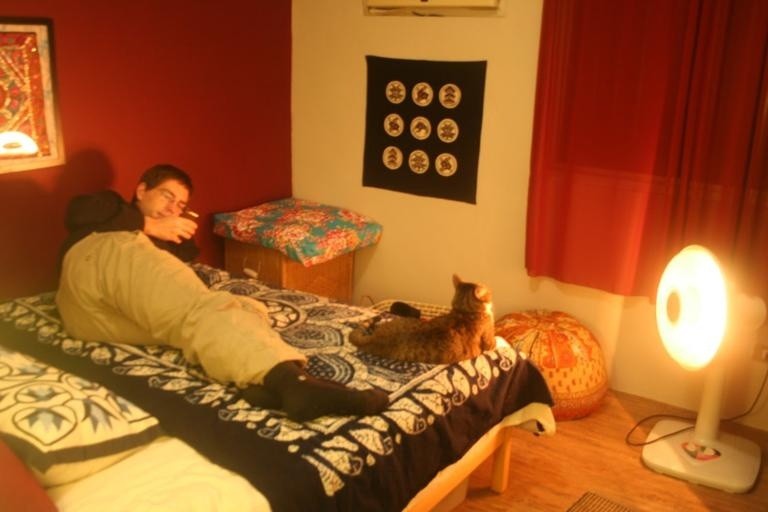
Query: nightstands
223,237,356,305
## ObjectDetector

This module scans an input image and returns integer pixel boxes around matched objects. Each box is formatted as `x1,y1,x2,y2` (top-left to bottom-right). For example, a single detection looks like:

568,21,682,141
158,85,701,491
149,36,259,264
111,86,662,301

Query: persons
56,163,389,421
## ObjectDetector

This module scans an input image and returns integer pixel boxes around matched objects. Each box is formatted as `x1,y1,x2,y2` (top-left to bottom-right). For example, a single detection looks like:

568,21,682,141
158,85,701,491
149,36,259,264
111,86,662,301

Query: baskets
370,300,451,319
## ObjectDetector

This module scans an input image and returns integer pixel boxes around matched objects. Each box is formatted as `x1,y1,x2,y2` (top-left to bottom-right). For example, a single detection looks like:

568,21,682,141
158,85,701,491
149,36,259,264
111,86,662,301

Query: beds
0,258,531,512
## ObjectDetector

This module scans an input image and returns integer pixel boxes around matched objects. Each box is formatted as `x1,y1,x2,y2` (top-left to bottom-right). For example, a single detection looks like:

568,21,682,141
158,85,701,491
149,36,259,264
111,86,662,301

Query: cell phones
181,208,200,224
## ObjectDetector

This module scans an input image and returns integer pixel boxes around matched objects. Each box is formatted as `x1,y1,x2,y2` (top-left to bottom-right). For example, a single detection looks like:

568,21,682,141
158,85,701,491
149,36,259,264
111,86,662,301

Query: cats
348,273,497,365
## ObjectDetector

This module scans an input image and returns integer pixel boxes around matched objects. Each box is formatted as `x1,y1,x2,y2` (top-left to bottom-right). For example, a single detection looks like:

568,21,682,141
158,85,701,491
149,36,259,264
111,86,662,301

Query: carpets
565,490,647,512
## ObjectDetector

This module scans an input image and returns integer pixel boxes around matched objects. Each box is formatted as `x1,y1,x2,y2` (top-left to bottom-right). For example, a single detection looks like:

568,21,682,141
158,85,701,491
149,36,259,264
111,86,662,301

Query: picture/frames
0,17,67,176
